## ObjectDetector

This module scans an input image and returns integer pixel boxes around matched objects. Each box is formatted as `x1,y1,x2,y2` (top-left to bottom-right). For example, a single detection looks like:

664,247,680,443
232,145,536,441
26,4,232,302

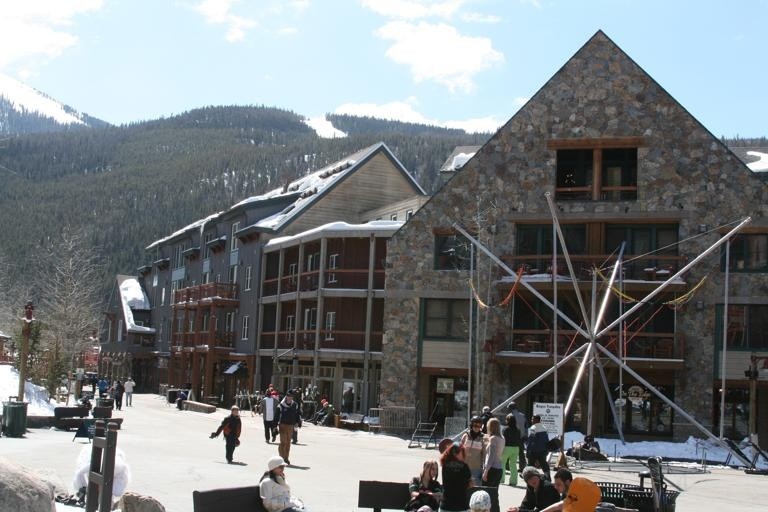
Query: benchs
357,479,500,512
175,399,216,414
340,412,365,432
191,484,273,512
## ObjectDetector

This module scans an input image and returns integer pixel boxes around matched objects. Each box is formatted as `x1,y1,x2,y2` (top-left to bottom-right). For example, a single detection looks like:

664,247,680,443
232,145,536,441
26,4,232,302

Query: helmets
470,414,483,427
482,405,490,412
507,401,518,408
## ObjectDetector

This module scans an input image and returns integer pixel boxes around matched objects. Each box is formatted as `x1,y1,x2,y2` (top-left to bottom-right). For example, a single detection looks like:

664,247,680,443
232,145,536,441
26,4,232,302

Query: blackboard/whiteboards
83,420,108,439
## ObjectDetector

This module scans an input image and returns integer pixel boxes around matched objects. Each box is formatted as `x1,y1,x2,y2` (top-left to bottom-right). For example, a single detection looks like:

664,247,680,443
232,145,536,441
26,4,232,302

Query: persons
175,389,187,411
215,406,242,464
312,399,336,427
259,456,305,511
259,384,303,466
343,387,355,413
73,374,136,410
407,402,601,512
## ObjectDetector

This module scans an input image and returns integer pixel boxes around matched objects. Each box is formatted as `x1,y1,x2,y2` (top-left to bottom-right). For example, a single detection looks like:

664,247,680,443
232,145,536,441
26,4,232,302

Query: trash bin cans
1,396,29,438
588,481,678,512
165,389,188,403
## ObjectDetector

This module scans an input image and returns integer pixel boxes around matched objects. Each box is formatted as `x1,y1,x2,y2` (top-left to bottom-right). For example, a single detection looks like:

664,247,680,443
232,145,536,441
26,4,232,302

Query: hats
522,465,541,482
469,489,492,510
267,456,289,471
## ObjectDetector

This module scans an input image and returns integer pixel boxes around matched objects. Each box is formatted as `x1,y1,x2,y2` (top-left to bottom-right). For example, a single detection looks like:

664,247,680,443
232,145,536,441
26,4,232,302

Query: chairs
514,334,675,359
